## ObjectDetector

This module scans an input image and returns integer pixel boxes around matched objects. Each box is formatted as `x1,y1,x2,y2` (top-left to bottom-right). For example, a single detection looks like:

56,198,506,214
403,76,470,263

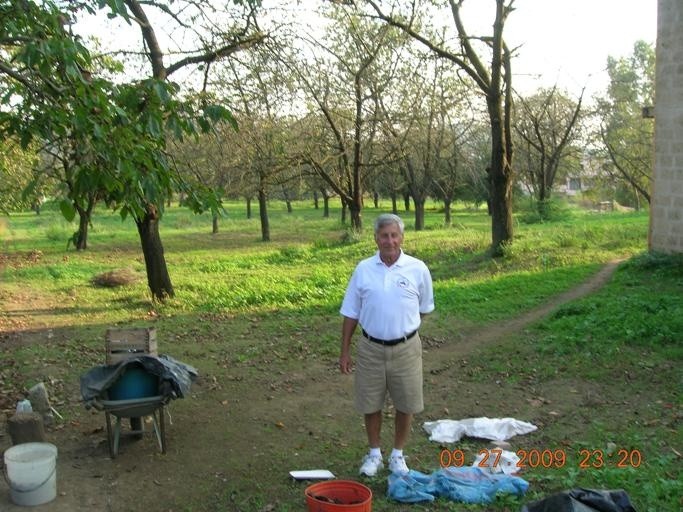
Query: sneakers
359,455,384,476
389,455,410,473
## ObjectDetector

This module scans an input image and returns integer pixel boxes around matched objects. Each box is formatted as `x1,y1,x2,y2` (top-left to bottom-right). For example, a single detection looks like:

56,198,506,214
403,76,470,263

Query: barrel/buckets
2,443,60,505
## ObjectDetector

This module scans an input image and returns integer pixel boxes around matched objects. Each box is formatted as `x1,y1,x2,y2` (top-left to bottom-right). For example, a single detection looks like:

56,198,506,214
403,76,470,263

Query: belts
360,329,417,348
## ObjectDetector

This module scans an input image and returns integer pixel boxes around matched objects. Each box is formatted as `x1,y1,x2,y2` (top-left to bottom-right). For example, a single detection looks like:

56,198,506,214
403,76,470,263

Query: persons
340,214,435,477
